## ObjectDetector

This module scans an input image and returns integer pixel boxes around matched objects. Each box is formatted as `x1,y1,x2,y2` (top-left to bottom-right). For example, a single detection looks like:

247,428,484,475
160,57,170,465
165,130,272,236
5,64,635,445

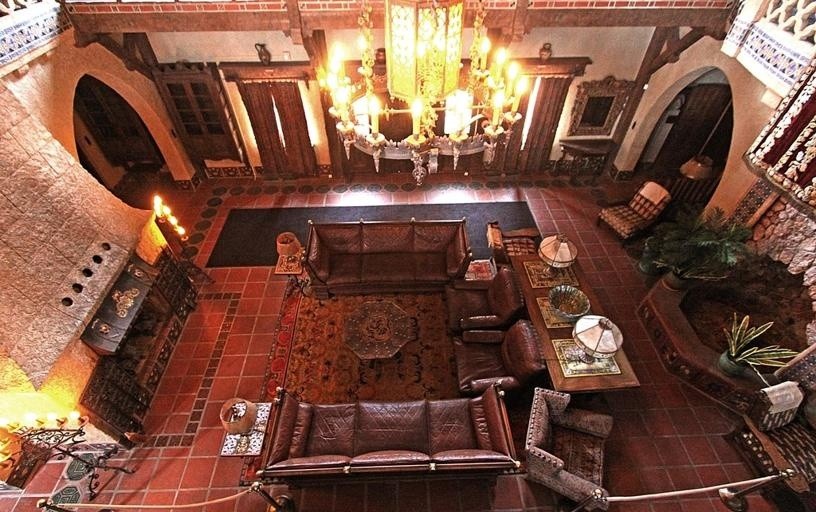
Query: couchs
252,380,522,480
521,382,612,511
449,318,546,396
301,217,474,301
482,219,540,263
441,265,526,334
721,376,815,510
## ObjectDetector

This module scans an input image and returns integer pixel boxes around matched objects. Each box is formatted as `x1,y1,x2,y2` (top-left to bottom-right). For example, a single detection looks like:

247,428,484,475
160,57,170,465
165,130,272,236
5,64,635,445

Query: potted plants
635,203,758,293
712,310,803,382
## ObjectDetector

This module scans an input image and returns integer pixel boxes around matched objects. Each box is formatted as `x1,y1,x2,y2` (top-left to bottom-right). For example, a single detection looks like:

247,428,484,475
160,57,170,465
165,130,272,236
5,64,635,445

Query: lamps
538,232,578,278
314,0,539,189
275,230,303,264
672,153,714,205
569,312,627,364
218,397,266,445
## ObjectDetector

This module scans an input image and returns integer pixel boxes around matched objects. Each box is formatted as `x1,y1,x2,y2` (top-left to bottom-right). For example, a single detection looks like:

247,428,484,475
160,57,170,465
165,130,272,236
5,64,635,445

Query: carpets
201,201,545,271
233,273,627,492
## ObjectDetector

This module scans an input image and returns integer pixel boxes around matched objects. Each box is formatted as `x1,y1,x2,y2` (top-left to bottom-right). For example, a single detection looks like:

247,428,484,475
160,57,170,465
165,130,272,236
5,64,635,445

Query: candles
321,37,531,141
0,410,97,454
151,193,188,249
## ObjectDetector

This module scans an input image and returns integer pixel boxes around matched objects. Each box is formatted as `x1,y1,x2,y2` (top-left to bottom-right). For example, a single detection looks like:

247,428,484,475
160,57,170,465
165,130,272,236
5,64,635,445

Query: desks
510,253,642,410
216,400,274,472
274,245,308,299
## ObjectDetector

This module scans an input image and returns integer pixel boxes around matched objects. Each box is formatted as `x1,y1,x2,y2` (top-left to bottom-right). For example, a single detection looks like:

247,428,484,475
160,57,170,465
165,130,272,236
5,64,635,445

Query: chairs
595,177,673,249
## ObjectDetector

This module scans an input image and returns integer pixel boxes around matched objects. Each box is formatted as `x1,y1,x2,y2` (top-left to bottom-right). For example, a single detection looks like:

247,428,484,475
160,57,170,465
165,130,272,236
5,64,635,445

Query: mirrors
566,75,638,137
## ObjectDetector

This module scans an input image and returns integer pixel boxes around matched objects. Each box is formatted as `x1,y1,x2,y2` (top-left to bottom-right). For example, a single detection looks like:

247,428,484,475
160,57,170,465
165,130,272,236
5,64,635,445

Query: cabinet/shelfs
147,59,246,165
547,136,618,186
